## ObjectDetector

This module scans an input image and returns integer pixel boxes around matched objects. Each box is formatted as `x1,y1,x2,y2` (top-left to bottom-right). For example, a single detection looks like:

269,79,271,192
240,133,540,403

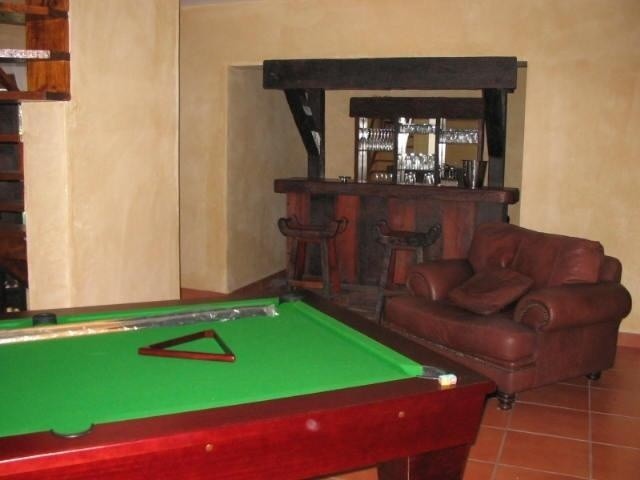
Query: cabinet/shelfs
355,117,479,189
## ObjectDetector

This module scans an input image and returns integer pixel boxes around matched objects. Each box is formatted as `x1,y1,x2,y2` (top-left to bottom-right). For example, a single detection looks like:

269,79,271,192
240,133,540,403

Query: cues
0,305,271,346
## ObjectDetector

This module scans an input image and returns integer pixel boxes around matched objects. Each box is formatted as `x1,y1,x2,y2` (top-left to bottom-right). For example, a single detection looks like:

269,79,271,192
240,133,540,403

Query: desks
1,288,498,480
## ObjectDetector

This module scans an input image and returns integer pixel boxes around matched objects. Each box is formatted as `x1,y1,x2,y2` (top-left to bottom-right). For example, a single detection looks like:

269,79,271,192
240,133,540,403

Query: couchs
386,220,631,410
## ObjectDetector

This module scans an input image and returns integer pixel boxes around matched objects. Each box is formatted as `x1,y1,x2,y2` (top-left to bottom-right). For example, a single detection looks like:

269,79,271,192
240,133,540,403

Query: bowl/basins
463,159,488,190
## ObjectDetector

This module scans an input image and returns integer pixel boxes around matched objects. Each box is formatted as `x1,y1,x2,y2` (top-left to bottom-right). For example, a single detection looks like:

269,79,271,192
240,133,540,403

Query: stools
375,225,443,290
277,215,348,290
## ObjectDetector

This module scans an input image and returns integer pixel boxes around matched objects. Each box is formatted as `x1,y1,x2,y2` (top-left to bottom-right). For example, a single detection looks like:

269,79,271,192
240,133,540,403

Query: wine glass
358,127,394,151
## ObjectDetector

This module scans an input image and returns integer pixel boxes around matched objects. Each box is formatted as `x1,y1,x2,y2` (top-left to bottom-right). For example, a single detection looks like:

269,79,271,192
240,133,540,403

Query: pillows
448,260,534,318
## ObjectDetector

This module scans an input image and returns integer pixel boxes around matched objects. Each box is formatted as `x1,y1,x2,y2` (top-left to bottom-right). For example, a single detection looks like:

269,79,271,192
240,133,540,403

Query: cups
403,171,435,185
398,151,435,170
441,162,456,181
370,171,393,183
399,123,436,133
438,128,479,144
